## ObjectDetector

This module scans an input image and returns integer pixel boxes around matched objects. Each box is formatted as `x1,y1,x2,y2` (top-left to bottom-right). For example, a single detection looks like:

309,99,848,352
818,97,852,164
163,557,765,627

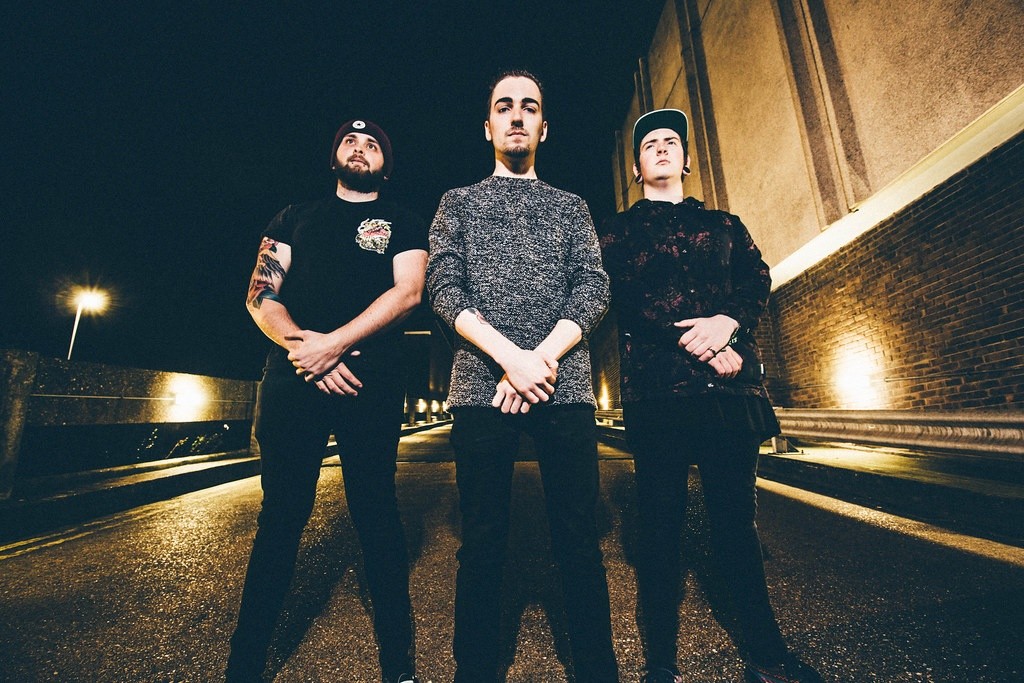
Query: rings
707,347,717,358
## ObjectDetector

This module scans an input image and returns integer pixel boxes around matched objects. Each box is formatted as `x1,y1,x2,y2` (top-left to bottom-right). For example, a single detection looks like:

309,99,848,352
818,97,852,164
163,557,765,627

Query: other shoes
741,657,823,683
641,661,683,683
382,673,419,683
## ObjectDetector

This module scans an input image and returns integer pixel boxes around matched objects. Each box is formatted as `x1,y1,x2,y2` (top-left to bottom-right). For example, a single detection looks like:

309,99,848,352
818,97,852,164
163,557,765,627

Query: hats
633,109,688,166
331,119,392,169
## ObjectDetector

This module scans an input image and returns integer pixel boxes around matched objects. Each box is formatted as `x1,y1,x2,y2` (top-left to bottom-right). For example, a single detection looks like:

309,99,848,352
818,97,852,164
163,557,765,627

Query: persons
597,107,823,682
224,116,432,683
422,65,621,683
